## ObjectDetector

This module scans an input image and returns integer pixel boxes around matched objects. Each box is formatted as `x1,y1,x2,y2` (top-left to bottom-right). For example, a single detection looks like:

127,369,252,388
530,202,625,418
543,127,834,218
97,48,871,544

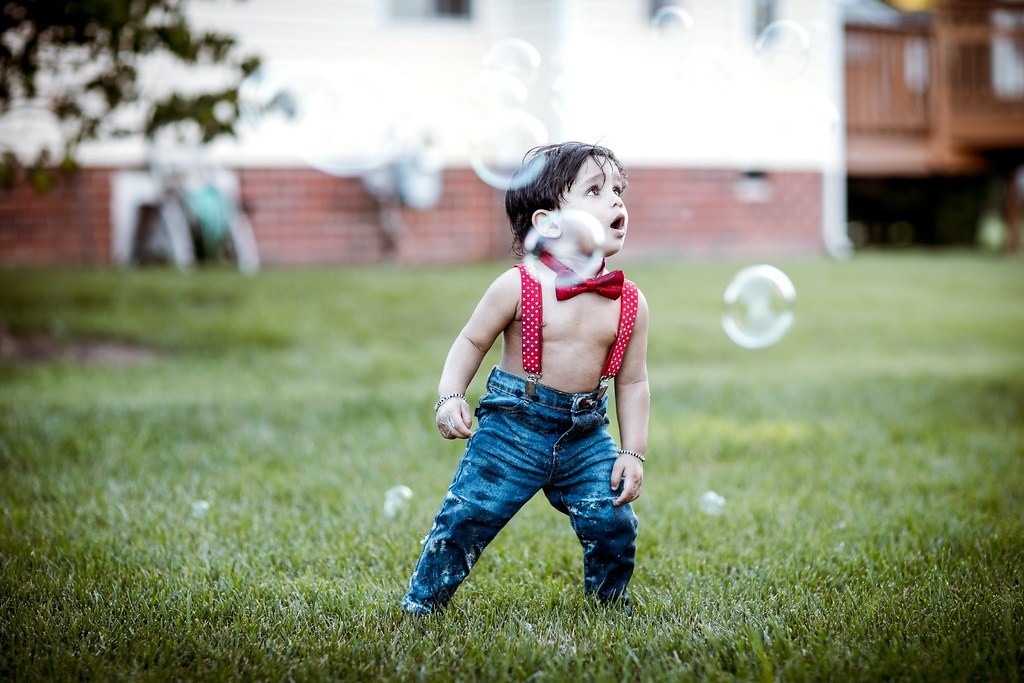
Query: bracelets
433,394,468,411
616,448,647,464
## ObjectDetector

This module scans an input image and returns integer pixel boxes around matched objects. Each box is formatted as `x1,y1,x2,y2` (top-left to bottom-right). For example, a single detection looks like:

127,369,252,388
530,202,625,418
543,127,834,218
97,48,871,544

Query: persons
397,141,649,622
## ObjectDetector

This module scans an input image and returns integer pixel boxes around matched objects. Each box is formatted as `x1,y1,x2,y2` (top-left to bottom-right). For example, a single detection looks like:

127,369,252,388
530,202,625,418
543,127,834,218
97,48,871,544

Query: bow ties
539,251,623,301
